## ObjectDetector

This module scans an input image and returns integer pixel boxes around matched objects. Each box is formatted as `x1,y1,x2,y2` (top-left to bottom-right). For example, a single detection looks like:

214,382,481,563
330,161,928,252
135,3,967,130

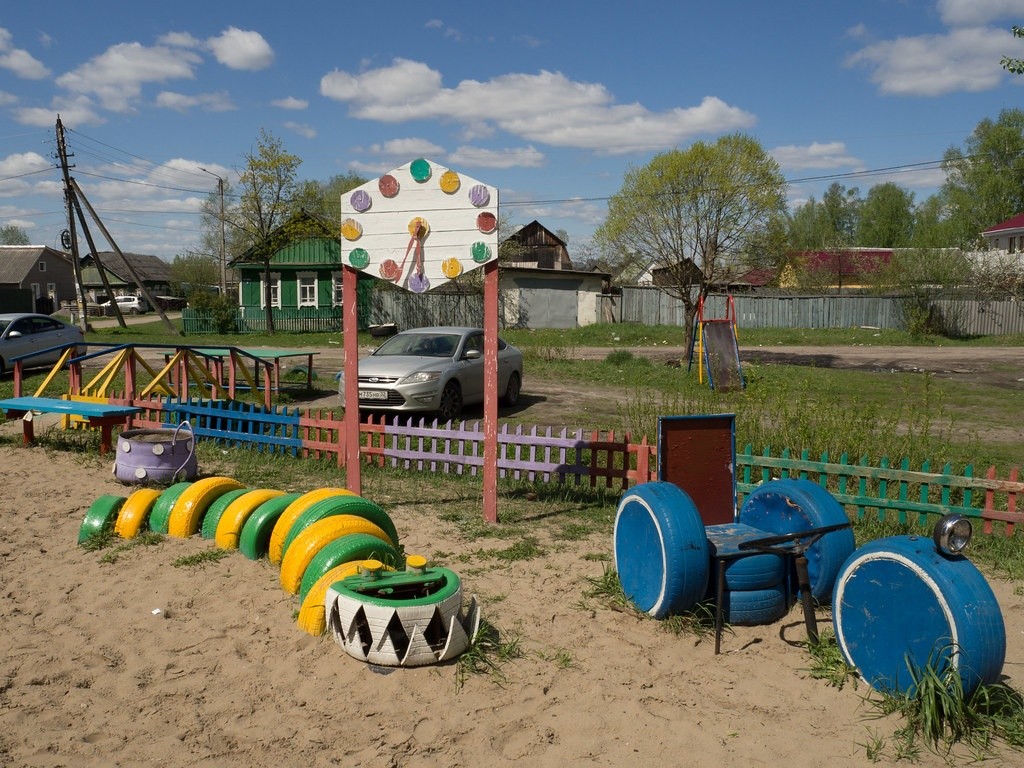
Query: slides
703,319,746,394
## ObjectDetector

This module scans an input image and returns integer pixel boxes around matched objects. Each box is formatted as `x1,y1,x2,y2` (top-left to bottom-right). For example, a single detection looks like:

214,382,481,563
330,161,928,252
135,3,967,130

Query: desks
156,349,321,402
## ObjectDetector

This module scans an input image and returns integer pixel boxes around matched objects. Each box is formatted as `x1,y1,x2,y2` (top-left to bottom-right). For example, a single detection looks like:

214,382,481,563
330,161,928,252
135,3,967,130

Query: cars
0,313,88,377
101,295,149,315
334,325,524,422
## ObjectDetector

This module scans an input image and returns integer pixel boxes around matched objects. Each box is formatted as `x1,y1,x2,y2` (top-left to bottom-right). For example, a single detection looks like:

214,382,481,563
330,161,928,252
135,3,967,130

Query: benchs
0,396,142,455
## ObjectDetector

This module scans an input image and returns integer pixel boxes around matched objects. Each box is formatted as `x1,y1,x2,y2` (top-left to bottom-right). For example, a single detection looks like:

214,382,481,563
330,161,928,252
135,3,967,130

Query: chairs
657,413,795,626
415,336,433,353
